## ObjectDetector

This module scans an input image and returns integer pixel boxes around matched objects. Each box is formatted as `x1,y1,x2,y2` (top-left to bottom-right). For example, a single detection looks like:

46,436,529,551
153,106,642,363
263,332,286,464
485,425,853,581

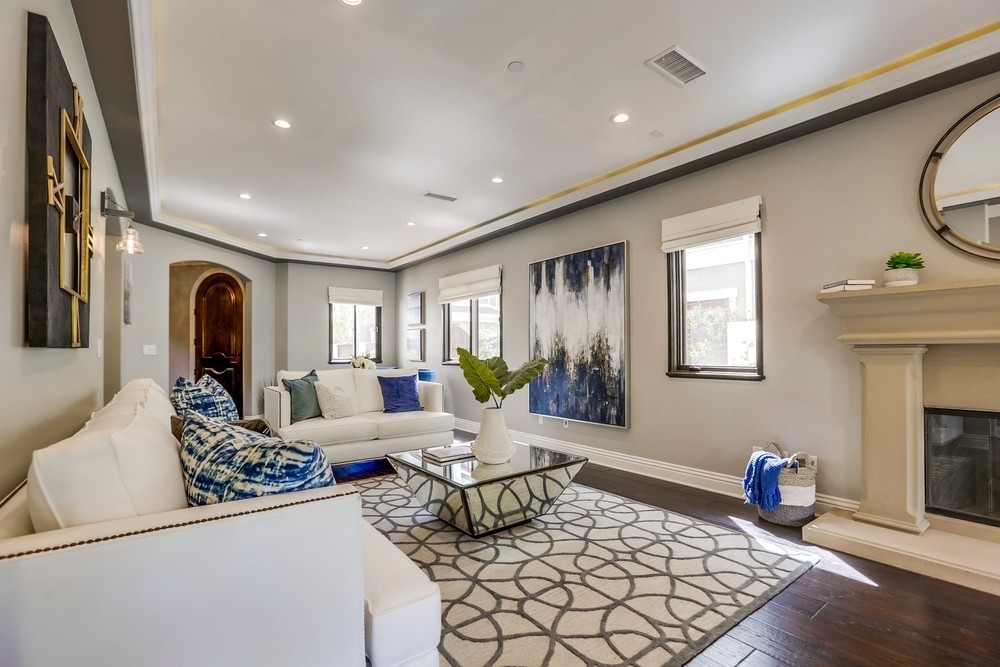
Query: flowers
351,353,375,369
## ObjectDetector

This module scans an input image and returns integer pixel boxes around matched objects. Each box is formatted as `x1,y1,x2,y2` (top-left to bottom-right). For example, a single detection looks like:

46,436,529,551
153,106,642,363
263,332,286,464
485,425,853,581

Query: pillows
377,374,424,414
170,373,336,508
313,381,355,420
280,369,322,425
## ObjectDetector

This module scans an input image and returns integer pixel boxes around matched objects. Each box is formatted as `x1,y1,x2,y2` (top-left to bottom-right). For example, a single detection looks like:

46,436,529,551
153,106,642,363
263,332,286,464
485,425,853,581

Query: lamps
101,190,145,256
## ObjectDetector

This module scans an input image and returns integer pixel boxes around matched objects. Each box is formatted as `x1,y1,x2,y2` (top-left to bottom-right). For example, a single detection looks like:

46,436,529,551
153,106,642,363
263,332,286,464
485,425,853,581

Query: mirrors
919,93,1000,262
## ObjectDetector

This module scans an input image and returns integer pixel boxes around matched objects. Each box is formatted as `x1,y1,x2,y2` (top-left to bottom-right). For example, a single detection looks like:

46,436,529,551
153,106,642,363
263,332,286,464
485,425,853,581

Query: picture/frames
528,241,631,431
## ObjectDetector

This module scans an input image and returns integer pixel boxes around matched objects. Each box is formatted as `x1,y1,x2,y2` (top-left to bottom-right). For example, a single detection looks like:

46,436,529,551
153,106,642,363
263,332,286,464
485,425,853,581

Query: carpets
336,472,822,667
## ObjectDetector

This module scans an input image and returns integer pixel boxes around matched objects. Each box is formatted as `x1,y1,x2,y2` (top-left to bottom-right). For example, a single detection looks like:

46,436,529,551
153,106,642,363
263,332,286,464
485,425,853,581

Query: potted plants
456,346,550,465
882,252,925,286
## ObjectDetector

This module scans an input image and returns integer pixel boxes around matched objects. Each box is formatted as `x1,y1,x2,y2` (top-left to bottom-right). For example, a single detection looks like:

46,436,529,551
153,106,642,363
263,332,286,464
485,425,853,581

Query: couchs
262,369,455,463
0,378,441,667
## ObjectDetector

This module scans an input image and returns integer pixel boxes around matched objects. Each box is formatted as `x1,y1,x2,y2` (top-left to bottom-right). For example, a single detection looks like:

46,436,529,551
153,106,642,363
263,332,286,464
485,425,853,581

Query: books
422,457,477,467
820,279,875,294
422,444,474,462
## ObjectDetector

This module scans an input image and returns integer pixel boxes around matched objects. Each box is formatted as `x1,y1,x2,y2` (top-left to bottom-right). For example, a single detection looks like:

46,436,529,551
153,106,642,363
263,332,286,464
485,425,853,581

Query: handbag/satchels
755,441,818,528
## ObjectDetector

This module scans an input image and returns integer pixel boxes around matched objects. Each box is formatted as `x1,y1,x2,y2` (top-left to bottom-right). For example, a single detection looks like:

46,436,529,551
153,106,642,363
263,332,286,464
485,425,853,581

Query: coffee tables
384,439,588,540
